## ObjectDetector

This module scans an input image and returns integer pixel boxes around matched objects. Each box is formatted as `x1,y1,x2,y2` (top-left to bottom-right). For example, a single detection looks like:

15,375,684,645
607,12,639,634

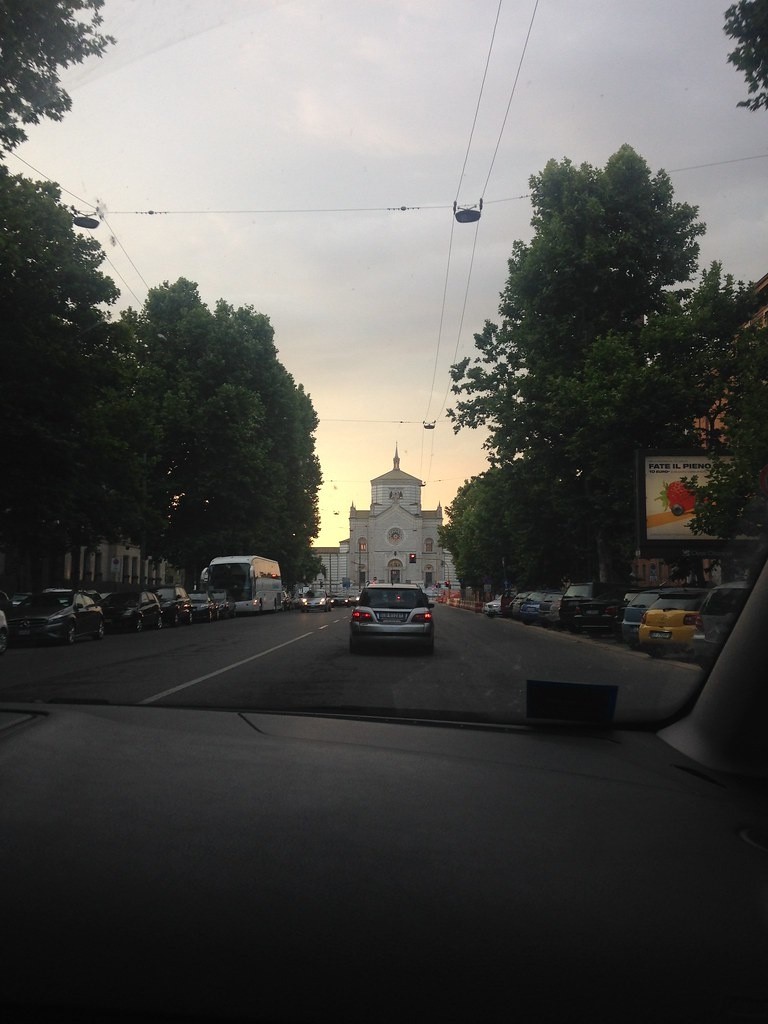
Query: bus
201,555,283,615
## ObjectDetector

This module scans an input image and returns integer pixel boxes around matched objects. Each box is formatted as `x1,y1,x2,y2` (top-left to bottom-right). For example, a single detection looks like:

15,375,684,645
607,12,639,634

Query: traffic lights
444,581,449,587
436,582,439,589
409,554,417,563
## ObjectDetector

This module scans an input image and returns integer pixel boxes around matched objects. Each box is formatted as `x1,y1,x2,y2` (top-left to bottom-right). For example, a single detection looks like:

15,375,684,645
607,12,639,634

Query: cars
484,583,755,672
0,588,237,659
349,583,436,652
282,590,362,614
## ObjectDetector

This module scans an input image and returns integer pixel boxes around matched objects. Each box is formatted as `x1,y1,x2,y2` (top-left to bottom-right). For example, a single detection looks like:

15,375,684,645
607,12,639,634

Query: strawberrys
654,481,708,512
